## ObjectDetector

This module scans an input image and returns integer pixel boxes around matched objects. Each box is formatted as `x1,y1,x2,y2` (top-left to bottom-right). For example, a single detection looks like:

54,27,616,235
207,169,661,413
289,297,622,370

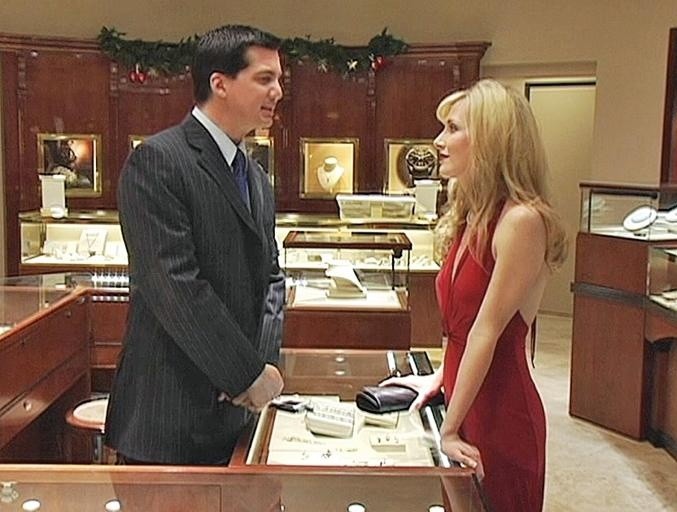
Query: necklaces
323,171,334,182
85,232,98,251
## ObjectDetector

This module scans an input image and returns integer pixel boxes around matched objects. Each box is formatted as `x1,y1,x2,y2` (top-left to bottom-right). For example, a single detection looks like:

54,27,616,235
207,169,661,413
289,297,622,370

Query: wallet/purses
354,377,446,412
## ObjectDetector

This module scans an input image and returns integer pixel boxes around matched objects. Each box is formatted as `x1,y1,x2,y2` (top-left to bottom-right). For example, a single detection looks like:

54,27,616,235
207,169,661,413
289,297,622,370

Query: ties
230,151,251,212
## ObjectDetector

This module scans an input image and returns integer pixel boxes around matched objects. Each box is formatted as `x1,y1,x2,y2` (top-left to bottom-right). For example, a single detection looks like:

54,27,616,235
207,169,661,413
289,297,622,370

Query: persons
99,21,287,470
315,156,345,193
375,79,574,511
